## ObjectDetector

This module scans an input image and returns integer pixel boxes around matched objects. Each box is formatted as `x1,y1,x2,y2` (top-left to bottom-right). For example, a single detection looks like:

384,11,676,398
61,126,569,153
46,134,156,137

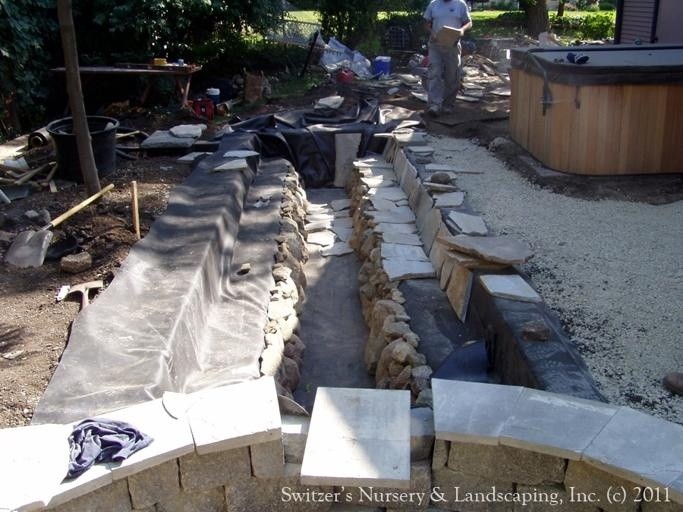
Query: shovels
68,280,104,312
4,182,115,269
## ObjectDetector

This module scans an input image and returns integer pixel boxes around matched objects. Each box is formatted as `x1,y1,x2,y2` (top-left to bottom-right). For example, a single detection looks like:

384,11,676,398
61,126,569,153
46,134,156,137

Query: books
431,25,464,49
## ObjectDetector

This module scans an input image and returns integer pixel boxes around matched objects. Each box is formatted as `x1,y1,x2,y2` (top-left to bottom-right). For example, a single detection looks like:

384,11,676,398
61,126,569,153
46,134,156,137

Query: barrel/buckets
206,88,221,107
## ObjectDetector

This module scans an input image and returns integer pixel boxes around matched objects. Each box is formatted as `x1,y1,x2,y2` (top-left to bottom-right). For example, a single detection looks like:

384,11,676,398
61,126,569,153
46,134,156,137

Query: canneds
178,59,184,66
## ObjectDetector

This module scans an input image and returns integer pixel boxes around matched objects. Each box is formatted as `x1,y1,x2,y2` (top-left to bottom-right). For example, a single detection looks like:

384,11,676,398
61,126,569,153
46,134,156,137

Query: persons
422,0,472,115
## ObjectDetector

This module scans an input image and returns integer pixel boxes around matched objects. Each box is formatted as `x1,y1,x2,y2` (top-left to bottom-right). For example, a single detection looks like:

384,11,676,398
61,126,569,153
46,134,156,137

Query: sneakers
427,102,455,117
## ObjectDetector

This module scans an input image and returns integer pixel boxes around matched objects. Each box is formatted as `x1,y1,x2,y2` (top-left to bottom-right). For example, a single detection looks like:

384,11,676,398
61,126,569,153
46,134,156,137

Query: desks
45,62,205,117
